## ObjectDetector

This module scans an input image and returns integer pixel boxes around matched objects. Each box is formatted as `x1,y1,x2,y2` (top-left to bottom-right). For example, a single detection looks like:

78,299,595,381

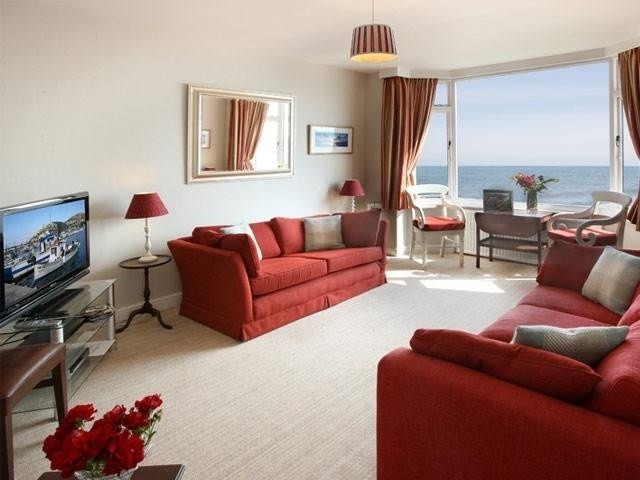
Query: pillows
332,208,382,247
535,237,640,293
270,217,305,255
220,223,263,261
409,328,604,402
591,318,640,424
199,229,260,272
617,284,640,325
191,225,233,243
249,221,281,259
581,244,640,316
304,215,346,252
509,324,630,363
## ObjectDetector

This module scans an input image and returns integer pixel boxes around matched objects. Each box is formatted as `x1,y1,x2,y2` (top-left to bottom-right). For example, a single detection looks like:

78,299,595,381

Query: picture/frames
483,189,513,211
307,124,354,155
201,129,211,149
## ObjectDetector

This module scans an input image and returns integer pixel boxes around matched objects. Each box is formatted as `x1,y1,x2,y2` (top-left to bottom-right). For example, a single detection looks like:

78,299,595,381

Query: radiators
455,207,545,265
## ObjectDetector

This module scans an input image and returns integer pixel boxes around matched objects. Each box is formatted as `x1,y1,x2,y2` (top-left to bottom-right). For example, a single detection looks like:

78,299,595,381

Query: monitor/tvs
0,191,91,328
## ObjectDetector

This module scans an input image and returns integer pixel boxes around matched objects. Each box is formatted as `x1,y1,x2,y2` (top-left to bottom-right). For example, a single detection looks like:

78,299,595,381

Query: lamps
125,192,169,263
339,179,366,212
348,1,398,63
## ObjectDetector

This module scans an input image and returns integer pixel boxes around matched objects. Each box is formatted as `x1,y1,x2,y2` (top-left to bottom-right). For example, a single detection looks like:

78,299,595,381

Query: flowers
42,393,163,478
512,172,560,195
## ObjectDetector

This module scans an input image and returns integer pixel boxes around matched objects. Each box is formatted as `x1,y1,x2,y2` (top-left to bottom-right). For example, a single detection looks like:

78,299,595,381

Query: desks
475,208,558,274
0,343,68,480
37,464,185,480
0,278,118,421
116,255,172,334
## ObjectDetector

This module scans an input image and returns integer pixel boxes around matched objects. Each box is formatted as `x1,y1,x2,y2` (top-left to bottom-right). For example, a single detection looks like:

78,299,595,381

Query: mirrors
186,84,296,183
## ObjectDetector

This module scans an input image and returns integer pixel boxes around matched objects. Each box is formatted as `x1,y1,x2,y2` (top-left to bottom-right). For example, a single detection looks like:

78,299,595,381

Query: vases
527,192,537,209
74,467,139,480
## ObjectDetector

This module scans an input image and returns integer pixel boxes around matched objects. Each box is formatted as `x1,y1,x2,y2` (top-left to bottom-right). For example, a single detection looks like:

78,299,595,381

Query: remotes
18,310,69,321
14,320,62,329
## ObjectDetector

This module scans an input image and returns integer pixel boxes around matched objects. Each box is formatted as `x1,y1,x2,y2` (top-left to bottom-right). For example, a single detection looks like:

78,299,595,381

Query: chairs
545,191,632,249
405,184,466,269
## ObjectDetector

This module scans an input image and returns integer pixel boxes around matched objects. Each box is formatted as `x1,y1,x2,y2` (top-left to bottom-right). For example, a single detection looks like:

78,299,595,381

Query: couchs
376,237,640,480
167,208,389,343
